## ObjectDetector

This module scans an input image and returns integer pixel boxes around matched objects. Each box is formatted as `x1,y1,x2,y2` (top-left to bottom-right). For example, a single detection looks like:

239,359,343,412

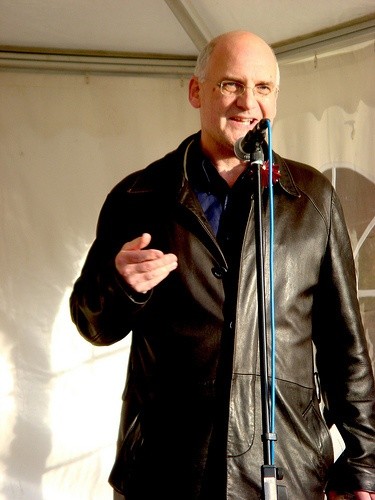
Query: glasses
199,76,276,97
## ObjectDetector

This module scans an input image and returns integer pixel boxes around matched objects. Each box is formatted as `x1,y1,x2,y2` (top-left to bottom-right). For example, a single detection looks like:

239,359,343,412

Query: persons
68,29,375,500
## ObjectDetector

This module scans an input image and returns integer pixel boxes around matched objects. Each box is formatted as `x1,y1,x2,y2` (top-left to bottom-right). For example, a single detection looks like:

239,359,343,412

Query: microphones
234,118,270,161
